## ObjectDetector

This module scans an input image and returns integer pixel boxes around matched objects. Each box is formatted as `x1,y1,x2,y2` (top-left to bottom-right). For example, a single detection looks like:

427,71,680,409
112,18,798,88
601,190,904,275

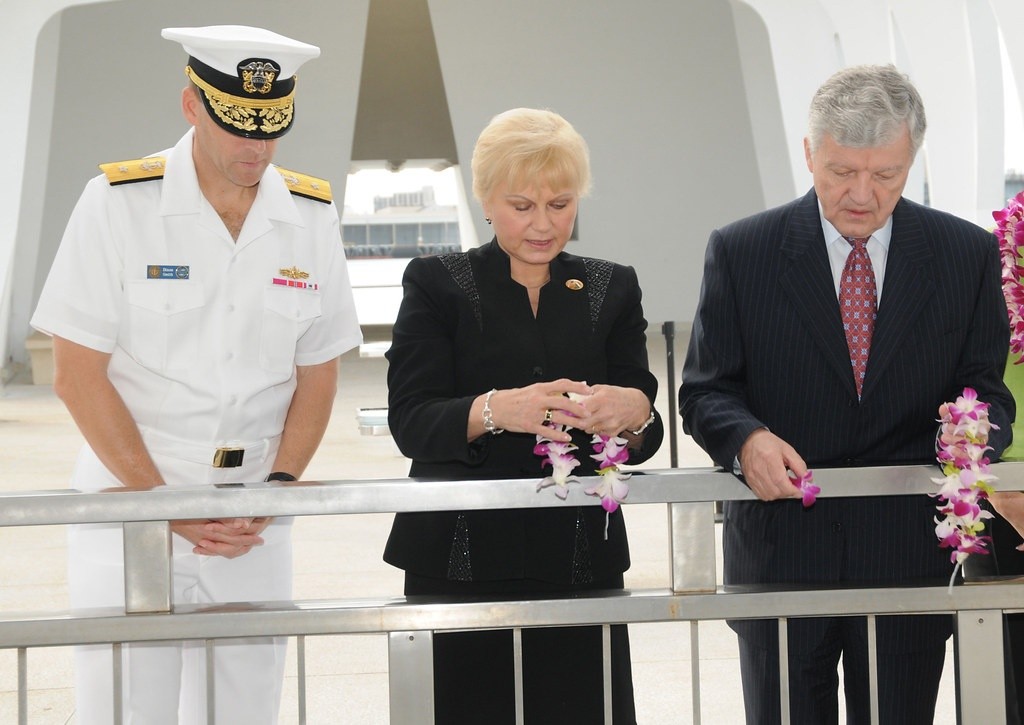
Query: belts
140,432,282,468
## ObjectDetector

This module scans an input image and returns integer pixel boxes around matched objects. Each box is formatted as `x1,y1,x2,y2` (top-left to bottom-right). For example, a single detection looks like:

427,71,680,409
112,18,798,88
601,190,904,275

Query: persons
680,67,1016,725
383,109,665,725
28,25,363,725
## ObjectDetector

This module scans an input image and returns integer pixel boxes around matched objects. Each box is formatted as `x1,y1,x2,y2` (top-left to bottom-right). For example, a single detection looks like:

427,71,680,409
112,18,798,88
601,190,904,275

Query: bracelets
264,472,296,481
482,389,504,434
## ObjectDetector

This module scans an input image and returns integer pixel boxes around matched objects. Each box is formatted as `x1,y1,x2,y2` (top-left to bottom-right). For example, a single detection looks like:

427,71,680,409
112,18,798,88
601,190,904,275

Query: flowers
928,388,991,565
789,471,820,507
992,190,1024,365
533,382,631,513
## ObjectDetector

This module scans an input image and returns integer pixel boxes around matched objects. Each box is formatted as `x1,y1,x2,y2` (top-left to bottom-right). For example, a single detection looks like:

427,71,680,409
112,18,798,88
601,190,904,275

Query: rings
545,410,552,421
592,426,594,434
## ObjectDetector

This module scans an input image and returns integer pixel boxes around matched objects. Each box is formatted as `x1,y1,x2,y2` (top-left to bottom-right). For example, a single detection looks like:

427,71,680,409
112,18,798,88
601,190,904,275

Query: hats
160,25,320,139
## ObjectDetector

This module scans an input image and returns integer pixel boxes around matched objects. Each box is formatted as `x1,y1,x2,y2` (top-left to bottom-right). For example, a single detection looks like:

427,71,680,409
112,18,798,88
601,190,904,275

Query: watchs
626,408,655,436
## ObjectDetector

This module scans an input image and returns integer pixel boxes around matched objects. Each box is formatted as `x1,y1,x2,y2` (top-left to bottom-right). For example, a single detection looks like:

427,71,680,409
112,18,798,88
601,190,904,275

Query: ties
838,235,877,404
